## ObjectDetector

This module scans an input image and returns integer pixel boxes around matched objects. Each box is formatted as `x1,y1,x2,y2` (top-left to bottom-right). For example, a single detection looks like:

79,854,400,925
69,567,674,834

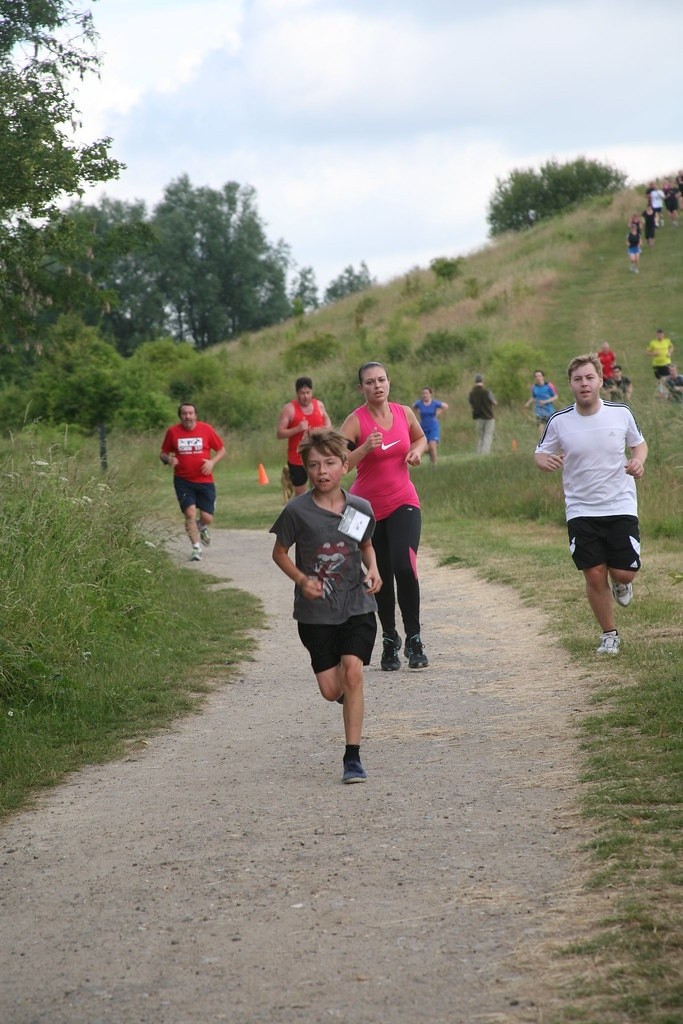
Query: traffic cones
510,439,517,452
257,463,270,486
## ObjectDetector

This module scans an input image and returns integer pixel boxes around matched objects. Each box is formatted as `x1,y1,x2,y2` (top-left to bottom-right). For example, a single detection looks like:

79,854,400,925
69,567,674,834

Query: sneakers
381,631,402,672
610,572,634,608
336,694,345,704
189,547,202,561
404,634,428,668
195,518,210,547
595,631,620,659
342,753,368,782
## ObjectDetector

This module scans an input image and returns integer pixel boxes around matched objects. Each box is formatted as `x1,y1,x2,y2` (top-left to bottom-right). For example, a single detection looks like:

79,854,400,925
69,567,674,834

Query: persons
160,403,226,561
642,203,656,248
412,387,449,466
604,365,634,402
645,170,683,227
534,354,647,651
645,329,673,399
598,342,616,389
628,213,643,235
468,374,497,452
277,377,332,498
626,224,643,274
525,369,558,433
270,429,383,784
664,364,683,403
341,362,426,672
